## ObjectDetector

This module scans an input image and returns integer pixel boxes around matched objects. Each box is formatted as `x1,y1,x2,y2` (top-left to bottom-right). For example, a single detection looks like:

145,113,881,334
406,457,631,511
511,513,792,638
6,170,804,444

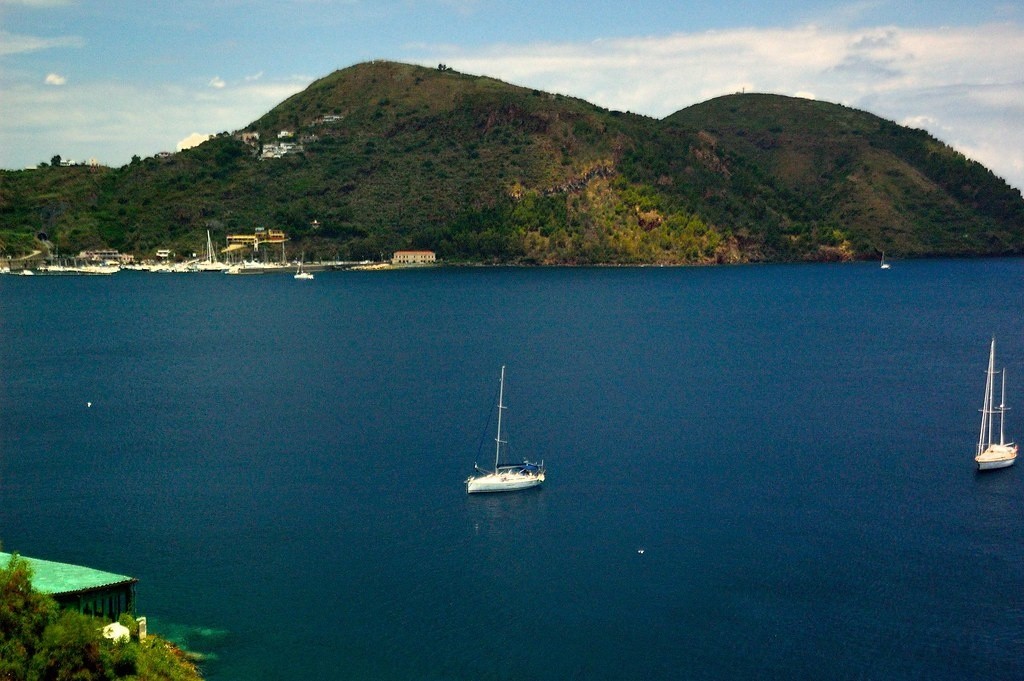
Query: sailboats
879,250,892,270
464,366,546,495
294,250,315,280
973,336,1019,471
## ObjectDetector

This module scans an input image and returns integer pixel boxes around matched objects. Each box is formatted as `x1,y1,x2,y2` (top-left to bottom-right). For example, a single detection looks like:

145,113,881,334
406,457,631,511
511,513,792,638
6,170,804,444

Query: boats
125,228,229,274
18,269,35,276
